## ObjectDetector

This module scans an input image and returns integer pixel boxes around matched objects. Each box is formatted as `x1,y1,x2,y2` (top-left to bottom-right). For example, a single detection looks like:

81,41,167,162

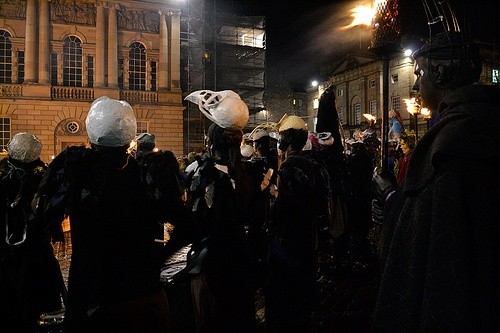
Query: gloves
371,168,396,200
371,198,385,225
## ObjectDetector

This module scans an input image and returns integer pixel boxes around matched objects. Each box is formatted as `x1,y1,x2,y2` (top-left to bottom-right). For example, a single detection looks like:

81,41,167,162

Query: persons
0,129,71,333
357,32,500,332
28,95,202,333
128,86,417,333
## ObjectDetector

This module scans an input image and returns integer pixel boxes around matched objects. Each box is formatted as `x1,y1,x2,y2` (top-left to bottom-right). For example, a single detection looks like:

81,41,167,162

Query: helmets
183,89,249,130
138,132,156,151
277,113,309,132
241,123,279,156
86,95,137,147
7,131,41,162
